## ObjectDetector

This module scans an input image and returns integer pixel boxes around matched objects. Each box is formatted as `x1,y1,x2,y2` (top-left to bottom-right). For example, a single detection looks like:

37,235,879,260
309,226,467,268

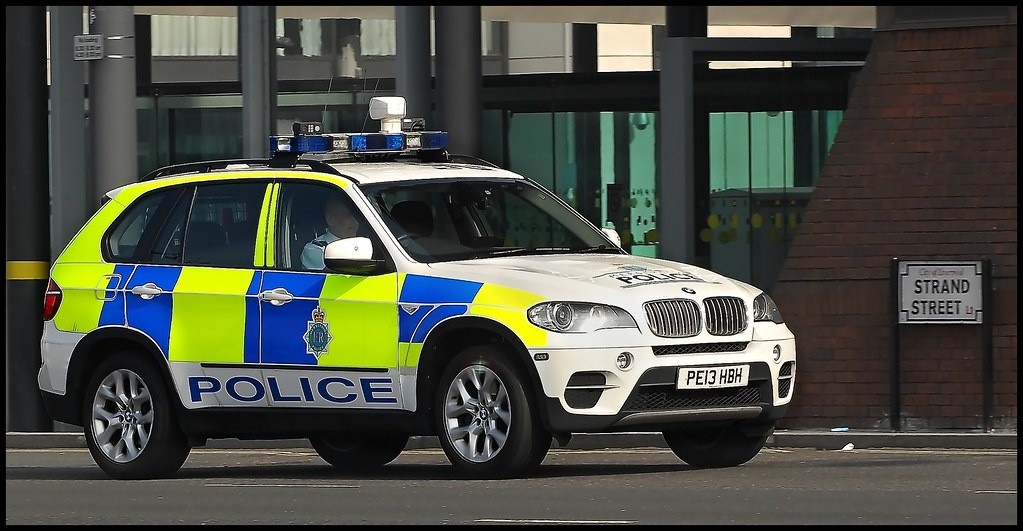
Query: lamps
631,113,650,130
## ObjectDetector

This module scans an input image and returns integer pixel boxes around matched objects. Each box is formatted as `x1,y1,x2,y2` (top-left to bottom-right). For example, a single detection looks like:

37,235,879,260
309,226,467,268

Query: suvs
35,95,797,480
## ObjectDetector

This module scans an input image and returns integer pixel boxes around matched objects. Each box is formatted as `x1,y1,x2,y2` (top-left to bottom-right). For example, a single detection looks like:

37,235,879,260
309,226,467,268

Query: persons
299,197,359,270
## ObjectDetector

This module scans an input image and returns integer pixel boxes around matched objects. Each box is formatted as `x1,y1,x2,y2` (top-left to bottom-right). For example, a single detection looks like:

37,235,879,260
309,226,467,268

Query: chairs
289,217,326,272
390,200,434,240
182,219,233,267
